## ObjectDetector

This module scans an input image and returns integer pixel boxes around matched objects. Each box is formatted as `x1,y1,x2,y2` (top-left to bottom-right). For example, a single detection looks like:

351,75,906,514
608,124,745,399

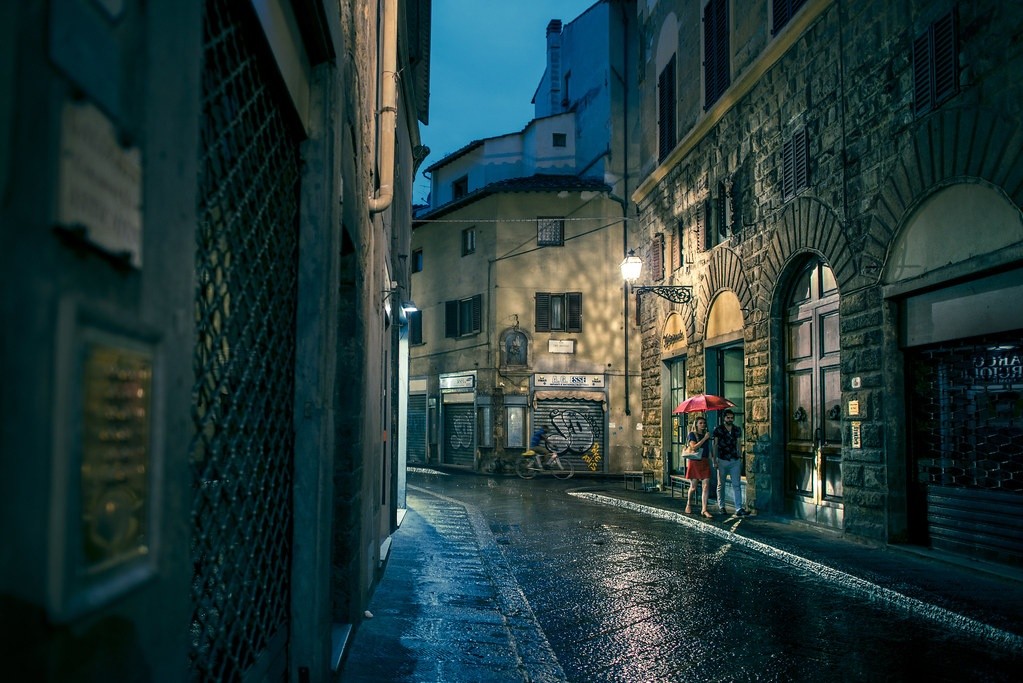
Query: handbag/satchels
681,431,703,460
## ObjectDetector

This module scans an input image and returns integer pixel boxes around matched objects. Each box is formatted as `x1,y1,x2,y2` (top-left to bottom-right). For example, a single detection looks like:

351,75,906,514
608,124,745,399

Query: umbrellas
672,389,738,441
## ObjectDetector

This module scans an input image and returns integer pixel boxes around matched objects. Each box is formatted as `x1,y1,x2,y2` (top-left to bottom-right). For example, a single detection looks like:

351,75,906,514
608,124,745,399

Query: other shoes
718,507,727,514
736,508,752,515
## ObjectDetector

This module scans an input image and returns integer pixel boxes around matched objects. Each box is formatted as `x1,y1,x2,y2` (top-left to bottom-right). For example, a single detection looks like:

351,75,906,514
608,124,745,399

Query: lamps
400,300,418,313
618,248,643,287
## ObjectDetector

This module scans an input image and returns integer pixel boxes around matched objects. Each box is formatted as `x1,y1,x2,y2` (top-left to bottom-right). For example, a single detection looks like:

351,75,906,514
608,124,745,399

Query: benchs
624,470,655,492
670,474,710,505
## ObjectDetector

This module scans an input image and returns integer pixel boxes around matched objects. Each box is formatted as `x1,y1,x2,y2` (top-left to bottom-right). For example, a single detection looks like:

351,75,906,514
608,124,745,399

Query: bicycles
516,444,575,480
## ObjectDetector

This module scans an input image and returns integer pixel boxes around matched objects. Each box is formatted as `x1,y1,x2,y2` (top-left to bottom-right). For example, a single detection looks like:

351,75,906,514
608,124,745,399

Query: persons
712,408,752,517
529,424,558,470
684,416,718,518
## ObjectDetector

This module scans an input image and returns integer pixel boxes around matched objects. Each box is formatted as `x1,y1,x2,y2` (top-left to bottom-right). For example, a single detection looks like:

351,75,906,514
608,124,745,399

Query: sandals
701,510,715,518
685,506,693,514
